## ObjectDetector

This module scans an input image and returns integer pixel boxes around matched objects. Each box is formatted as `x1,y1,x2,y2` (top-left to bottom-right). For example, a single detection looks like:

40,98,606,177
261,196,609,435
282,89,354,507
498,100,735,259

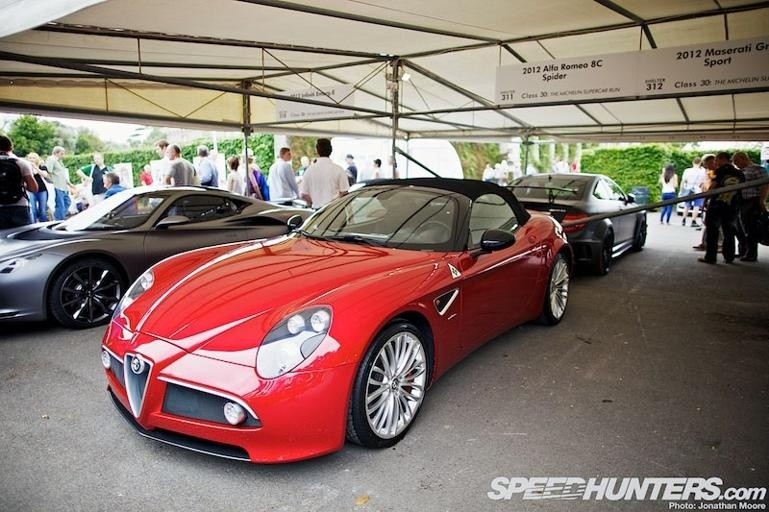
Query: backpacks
0,156,31,206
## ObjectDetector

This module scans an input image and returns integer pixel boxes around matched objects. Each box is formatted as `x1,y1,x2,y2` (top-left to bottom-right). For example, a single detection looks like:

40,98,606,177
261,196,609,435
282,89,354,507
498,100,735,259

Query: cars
495,170,649,276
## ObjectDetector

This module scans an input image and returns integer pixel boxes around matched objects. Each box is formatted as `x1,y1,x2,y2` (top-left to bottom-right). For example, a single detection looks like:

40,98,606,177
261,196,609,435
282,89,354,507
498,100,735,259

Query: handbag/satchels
249,185,261,195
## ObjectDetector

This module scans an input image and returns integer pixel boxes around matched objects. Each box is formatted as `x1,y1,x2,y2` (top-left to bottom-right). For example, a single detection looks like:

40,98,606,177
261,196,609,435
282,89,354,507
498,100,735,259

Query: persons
480,154,580,184
657,150,769,264
1,133,398,229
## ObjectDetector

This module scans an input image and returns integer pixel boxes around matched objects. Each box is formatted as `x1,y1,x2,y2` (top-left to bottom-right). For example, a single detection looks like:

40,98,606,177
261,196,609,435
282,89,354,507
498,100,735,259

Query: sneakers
682,221,685,225
691,223,700,227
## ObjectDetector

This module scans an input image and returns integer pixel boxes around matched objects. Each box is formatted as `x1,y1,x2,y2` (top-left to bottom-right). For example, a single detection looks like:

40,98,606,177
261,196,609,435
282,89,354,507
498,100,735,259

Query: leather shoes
691,244,758,265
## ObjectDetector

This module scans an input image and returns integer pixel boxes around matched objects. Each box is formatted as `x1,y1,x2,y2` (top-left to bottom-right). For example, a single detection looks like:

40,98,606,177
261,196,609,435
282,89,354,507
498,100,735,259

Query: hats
237,148,255,159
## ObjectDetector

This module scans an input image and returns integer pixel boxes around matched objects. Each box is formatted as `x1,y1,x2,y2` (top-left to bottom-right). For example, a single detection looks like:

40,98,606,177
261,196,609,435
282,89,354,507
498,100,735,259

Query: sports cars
96,173,571,462
0,179,321,339
